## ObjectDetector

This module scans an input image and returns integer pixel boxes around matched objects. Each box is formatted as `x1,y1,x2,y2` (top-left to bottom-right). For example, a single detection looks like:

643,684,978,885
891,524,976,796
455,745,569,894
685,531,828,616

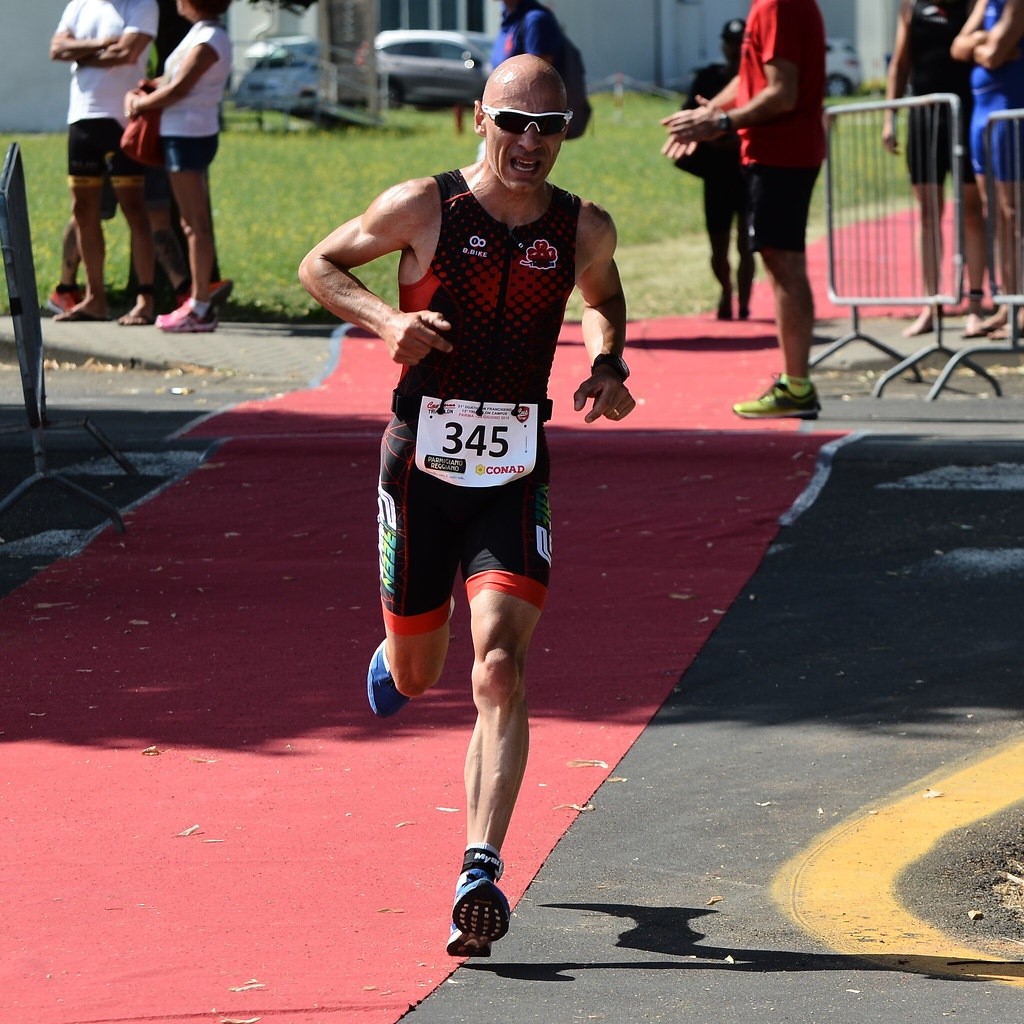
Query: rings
614,409,619,416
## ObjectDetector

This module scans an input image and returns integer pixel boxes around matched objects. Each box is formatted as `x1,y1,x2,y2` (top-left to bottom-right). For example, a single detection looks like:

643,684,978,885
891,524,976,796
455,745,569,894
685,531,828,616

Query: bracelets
719,112,731,130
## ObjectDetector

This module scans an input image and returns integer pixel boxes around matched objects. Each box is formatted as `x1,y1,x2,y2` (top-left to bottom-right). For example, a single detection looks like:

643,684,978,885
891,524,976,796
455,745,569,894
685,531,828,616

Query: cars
824,37,862,100
375,29,496,109
225,36,372,132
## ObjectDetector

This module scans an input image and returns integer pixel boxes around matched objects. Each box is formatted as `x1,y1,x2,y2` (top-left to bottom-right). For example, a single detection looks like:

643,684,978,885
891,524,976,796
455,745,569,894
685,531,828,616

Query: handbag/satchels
120,80,164,167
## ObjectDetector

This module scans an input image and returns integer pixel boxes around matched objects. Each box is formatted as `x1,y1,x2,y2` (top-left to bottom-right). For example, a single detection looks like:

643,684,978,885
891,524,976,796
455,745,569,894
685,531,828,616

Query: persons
491,0,560,66
659,0,829,419
47,0,234,334
673,19,755,320
880,0,1024,340
297,53,637,957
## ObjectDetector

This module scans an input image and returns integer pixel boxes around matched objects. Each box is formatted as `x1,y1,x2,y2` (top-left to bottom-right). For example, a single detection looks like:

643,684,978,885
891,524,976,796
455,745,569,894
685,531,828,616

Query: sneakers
46,282,83,312
734,373,820,421
447,867,509,957
155,301,218,333
176,279,233,306
367,639,413,716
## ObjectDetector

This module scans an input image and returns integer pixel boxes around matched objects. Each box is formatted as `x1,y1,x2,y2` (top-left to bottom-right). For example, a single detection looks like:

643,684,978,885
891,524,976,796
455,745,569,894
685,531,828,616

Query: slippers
118,311,155,326
54,308,114,321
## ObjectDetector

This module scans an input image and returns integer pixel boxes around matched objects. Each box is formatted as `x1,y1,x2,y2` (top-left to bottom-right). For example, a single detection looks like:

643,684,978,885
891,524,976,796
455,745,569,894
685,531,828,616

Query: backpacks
518,5,591,138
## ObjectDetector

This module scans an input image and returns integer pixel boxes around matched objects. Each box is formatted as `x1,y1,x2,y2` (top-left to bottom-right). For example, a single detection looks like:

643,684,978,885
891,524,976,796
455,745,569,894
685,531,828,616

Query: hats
723,19,746,38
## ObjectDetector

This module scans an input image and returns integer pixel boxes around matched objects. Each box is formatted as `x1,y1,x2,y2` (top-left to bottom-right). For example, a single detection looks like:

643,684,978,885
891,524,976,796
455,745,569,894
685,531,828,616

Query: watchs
590,353,630,382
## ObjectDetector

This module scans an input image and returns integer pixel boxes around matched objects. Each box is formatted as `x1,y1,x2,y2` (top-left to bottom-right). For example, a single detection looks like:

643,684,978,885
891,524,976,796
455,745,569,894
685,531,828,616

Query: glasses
481,105,573,135
